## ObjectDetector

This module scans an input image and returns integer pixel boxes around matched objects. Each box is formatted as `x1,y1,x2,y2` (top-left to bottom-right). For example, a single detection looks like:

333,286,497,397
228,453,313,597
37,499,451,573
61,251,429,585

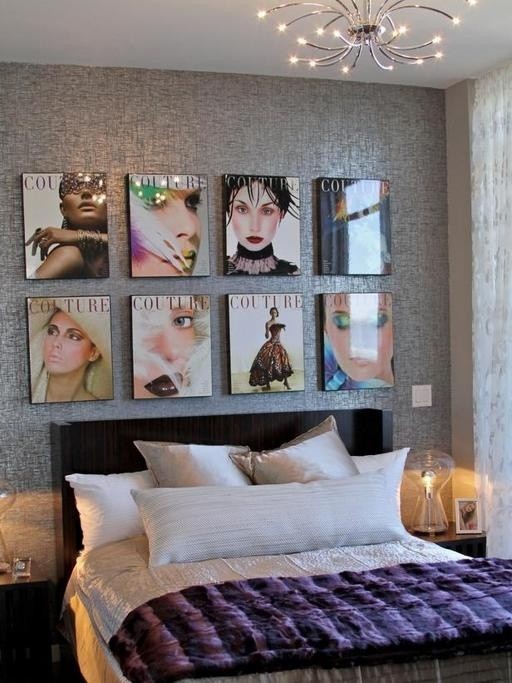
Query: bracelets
77,229,102,251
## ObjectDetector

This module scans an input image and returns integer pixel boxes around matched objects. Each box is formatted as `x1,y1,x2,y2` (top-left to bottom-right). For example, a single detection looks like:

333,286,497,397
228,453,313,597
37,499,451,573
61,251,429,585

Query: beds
51,407,512,683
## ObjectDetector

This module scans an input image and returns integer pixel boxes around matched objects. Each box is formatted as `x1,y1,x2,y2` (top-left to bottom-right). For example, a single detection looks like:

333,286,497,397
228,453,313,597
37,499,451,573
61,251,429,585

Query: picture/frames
11,557,32,578
456,498,482,534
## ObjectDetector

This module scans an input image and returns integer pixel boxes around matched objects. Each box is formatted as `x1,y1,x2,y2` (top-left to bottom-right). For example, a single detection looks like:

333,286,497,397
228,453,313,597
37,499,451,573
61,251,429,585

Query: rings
35,227,41,234
39,238,48,248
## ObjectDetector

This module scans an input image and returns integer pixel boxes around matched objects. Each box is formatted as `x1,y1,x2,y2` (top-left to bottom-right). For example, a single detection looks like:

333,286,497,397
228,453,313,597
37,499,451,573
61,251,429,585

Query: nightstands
406,521,486,557
0,573,51,683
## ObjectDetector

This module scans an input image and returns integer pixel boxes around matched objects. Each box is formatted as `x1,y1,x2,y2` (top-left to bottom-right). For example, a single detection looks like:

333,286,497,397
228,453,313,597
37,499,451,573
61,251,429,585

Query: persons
225,174,300,276
132,296,209,399
25,172,107,279
325,201,391,273
249,308,294,390
129,174,207,276
30,309,101,403
459,501,477,530
321,292,394,391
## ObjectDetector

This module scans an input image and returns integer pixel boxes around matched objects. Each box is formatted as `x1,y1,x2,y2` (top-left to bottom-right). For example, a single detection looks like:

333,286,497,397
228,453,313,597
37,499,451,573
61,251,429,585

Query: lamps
0,467,17,575
255,0,478,73
403,449,456,533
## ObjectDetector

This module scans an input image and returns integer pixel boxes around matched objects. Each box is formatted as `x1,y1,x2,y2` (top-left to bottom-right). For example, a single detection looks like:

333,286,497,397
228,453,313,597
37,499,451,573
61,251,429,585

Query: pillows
229,416,360,484
65,469,162,553
353,446,411,477
130,477,411,569
134,439,252,487
